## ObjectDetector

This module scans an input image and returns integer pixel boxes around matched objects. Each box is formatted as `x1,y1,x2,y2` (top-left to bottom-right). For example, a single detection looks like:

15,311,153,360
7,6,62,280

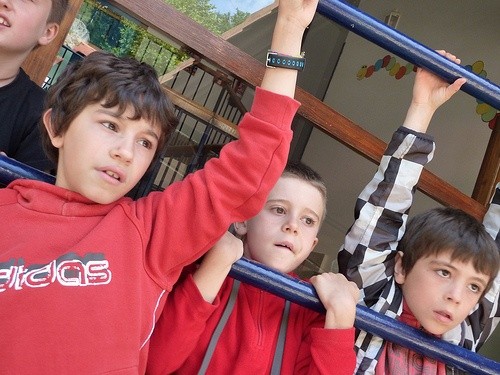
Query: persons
0,0,69,189
146,160,360,374
338,49,500,374
0,0,318,374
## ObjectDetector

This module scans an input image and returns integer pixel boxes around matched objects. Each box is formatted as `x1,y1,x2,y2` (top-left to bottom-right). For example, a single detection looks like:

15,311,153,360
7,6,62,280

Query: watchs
266,51,307,71
